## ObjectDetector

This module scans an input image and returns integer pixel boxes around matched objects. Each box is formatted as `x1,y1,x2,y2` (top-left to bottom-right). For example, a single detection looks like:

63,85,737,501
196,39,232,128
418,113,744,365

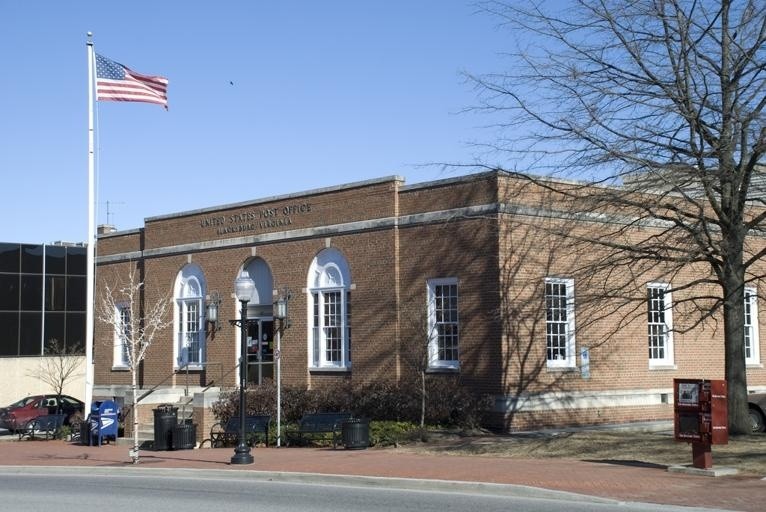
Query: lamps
206,294,222,340
273,285,288,338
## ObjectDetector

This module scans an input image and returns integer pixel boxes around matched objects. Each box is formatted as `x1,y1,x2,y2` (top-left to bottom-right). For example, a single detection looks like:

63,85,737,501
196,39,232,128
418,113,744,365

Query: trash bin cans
80,421,102,447
343,419,370,450
152,404,198,450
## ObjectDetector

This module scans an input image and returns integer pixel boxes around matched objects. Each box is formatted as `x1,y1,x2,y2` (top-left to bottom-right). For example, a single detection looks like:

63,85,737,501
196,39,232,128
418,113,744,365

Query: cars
747,394,766,432
0,395,85,434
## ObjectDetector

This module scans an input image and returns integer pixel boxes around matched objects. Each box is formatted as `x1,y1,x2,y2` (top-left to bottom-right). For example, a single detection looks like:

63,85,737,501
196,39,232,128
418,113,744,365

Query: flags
92,53,170,111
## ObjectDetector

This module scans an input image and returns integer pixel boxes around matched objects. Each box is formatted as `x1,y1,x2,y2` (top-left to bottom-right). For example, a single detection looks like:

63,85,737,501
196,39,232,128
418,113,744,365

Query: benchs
210,415,272,448
284,412,352,449
15,414,67,441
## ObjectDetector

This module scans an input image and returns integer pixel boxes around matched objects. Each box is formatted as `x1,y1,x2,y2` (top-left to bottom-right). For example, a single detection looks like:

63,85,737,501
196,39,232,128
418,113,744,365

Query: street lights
230,271,255,464
